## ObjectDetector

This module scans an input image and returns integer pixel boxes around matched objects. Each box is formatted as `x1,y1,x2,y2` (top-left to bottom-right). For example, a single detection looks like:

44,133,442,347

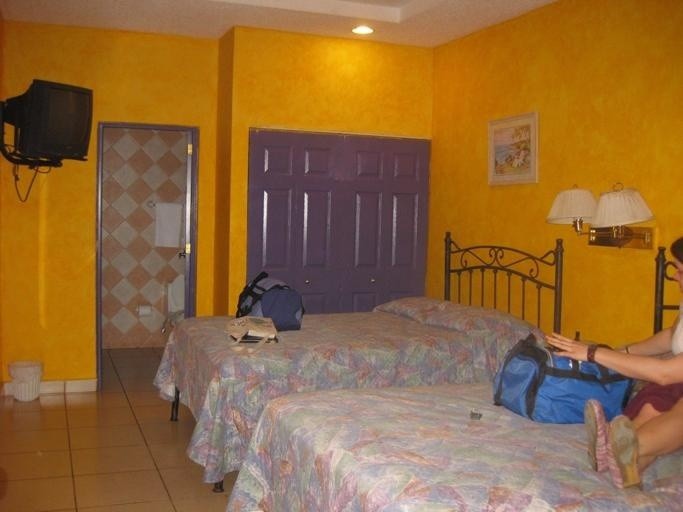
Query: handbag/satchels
493,331,634,424
224,271,305,354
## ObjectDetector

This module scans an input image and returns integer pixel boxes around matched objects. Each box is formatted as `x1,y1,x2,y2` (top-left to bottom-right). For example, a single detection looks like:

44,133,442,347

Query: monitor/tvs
3,79,93,161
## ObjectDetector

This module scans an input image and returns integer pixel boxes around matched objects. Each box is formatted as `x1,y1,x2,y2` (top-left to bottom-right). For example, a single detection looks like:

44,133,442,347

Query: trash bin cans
9,363,42,402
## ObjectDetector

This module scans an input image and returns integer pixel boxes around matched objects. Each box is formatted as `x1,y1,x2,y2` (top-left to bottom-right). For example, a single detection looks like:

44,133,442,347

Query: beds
224,246,683,512
170,232,564,493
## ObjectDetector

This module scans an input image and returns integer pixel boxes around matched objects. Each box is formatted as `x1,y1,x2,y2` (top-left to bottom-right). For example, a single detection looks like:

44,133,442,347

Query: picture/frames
487,112,538,185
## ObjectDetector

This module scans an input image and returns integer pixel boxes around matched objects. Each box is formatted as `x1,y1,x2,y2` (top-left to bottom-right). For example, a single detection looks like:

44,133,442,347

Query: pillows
425,301,540,336
373,296,441,325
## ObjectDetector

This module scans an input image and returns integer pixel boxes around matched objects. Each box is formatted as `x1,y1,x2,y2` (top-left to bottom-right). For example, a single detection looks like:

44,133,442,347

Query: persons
540,234,683,491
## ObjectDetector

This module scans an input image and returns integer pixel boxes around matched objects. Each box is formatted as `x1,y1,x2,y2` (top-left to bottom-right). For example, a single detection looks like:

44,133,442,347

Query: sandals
584,398,641,489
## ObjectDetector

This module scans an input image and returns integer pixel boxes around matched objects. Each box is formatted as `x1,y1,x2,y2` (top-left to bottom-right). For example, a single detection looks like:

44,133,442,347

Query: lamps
545,183,655,249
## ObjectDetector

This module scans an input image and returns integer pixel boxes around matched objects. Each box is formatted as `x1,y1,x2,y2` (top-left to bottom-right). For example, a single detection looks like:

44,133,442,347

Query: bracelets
585,342,611,364
623,345,631,355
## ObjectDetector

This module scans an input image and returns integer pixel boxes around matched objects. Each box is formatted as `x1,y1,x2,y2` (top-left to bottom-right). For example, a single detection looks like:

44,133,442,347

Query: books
247,330,276,341
231,333,278,344
